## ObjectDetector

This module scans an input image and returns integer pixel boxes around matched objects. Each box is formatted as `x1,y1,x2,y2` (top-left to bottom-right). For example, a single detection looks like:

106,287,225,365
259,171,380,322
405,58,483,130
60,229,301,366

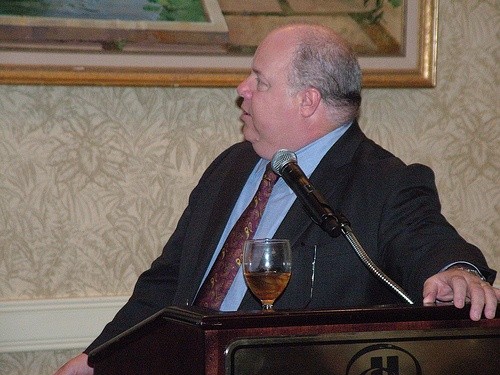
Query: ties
191,162,280,311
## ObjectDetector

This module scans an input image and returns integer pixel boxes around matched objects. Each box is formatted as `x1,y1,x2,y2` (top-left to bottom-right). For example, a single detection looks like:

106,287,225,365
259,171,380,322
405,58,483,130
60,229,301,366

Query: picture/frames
0,0,440,87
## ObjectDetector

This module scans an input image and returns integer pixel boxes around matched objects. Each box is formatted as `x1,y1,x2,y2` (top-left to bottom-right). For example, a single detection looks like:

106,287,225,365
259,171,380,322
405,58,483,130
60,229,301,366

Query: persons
52,23,500,375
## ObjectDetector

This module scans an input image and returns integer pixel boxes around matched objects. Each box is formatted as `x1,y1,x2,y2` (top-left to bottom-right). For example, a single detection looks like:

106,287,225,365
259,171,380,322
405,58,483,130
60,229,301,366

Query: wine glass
242,240,291,310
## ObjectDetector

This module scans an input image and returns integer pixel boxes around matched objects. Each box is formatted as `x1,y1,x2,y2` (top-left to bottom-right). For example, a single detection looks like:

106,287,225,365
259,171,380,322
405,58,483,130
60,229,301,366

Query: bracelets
456,266,485,279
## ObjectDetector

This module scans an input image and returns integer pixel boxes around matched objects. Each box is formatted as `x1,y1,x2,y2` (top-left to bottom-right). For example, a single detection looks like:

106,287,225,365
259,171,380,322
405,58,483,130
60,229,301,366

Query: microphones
271,148,341,238
